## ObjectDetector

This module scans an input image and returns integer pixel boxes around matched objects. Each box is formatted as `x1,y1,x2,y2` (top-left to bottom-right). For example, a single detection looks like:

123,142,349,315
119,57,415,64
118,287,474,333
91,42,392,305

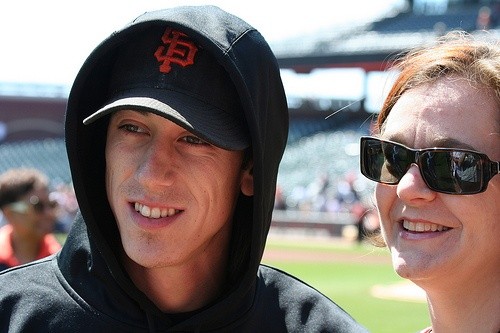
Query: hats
82,24,252,151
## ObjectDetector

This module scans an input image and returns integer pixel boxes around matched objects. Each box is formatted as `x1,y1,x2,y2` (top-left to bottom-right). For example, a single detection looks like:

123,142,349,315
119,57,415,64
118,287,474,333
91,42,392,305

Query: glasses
22,197,58,213
360,136,500,195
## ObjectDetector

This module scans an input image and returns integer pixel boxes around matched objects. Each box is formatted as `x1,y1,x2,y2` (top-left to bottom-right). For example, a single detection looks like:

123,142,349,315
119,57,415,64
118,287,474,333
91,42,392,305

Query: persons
451,151,482,193
360,30,500,333
0,5,371,333
0,167,62,273
